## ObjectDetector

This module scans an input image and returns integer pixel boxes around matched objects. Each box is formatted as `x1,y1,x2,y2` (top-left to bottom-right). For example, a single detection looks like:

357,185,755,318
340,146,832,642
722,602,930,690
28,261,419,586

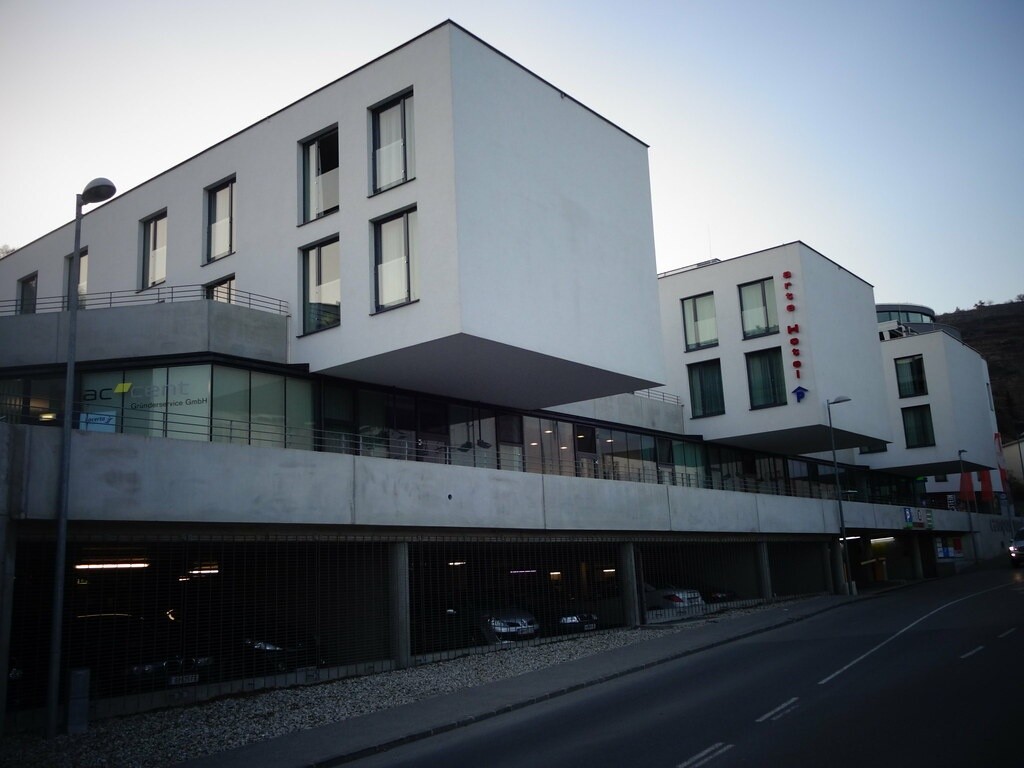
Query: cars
67,606,320,698
636,574,731,619
1009,526,1024,565
446,589,599,650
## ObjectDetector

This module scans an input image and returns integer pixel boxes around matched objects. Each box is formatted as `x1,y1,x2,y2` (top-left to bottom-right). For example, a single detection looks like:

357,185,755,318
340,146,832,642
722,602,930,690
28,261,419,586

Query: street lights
46,185,118,741
958,447,982,563
826,397,854,597
